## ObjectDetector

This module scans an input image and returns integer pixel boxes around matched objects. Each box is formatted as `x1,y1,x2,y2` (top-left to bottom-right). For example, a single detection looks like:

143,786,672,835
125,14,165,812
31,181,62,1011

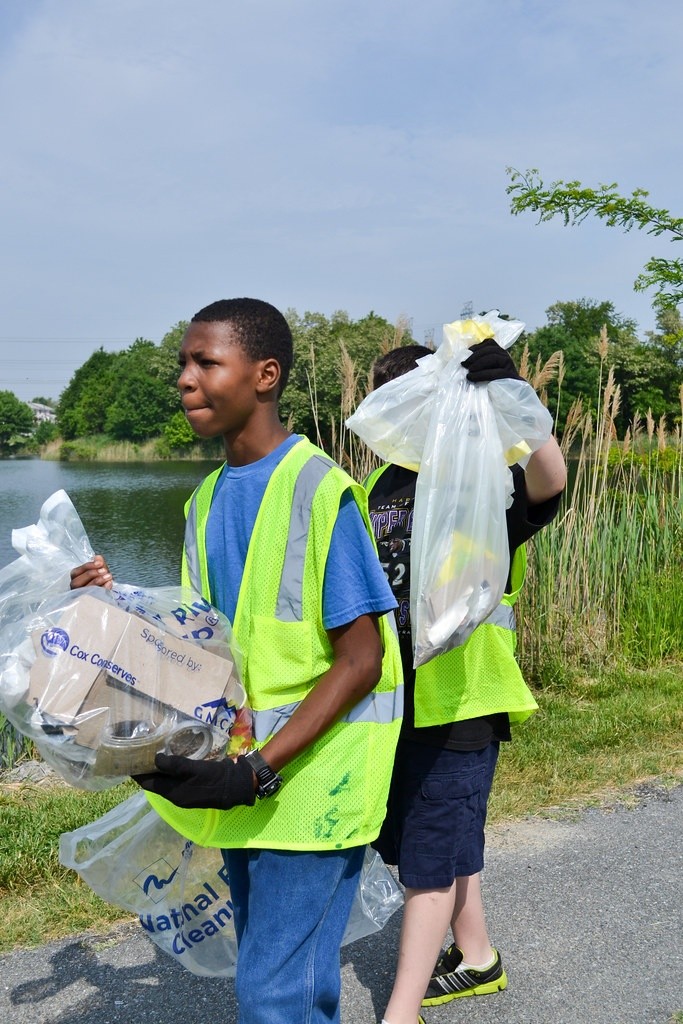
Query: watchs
243,747,284,802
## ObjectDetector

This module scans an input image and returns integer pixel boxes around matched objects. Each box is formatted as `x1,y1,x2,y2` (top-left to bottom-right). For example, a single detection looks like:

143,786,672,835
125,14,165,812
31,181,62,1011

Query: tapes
164,720,214,760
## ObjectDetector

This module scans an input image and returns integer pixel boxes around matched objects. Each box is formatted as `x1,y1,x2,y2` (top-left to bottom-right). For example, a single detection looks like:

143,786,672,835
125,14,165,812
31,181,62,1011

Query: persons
68,296,404,1023
358,336,566,1022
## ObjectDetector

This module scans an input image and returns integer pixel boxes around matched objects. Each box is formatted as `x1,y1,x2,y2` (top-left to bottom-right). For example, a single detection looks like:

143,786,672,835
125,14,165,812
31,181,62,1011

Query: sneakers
418,944,506,1006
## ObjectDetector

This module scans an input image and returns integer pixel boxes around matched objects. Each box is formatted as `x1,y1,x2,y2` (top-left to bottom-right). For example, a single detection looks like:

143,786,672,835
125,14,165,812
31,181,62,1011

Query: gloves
129,754,255,810
462,336,528,383
